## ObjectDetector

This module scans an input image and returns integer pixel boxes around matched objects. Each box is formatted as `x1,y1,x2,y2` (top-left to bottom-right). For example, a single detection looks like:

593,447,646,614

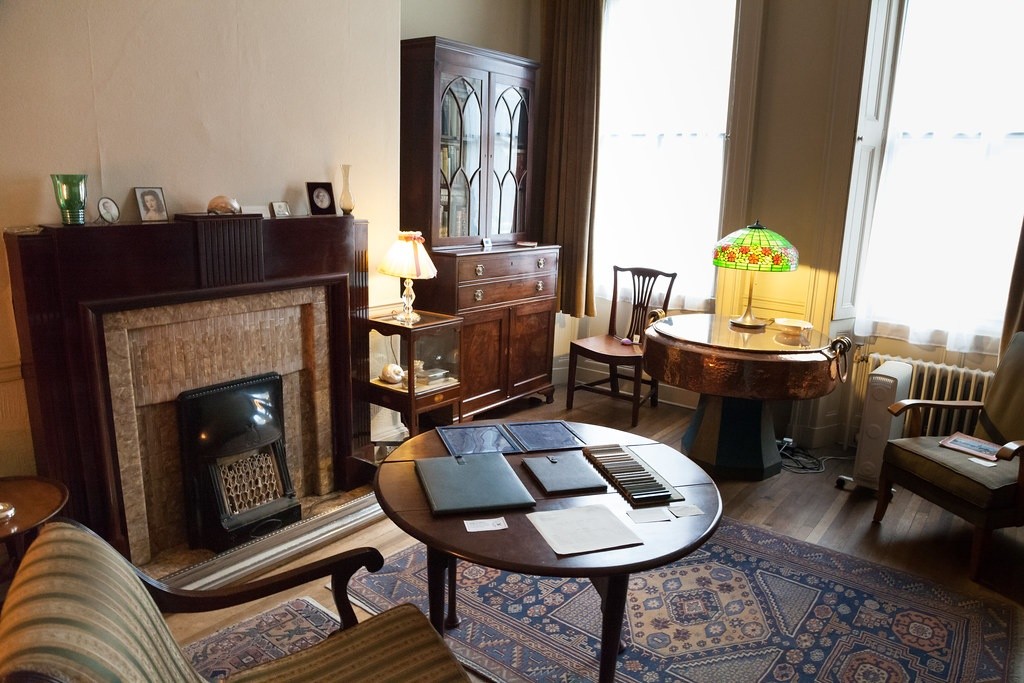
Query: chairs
872,333,1024,581
0,516,471,683
566,265,678,425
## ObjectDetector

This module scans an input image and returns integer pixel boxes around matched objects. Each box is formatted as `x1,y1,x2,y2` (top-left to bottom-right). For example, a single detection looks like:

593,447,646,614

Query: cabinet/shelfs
357,309,464,463
403,35,560,422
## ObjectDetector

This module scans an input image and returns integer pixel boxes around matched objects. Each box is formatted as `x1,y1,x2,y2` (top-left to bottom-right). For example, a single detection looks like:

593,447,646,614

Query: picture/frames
134,187,169,222
93,197,121,224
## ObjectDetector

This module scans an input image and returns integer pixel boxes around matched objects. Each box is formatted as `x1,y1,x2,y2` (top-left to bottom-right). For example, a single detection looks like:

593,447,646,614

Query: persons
103,201,118,222
141,190,168,220
316,191,329,209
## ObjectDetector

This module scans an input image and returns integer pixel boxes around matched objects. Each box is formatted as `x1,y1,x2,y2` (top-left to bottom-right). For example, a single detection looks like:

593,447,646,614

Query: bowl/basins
774,317,814,336
773,332,812,349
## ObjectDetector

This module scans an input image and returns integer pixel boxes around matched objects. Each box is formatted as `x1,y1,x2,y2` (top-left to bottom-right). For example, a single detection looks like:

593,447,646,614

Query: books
438,143,526,186
413,453,537,516
442,94,527,148
520,455,606,495
439,189,525,237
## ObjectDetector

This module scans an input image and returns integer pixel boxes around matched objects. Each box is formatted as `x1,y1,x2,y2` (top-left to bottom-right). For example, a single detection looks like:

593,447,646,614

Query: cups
50,173,87,225
0,501,19,536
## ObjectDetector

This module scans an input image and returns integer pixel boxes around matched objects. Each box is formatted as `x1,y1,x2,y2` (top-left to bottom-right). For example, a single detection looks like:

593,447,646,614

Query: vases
339,164,354,217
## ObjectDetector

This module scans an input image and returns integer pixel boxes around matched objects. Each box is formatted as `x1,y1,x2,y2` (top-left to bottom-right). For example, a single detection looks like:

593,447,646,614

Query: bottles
340,164,355,216
414,360,424,373
403,372,417,389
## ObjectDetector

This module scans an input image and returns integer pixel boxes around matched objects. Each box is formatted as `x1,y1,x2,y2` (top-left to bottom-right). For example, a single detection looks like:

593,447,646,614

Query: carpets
324,511,1023,682
178,595,342,682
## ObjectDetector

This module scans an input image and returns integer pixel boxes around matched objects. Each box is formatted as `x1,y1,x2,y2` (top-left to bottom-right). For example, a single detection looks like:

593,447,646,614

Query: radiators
863,354,994,444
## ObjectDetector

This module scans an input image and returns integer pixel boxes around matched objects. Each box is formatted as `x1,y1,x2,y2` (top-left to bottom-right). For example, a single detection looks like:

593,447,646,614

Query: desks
372,418,722,683
0,475,68,577
643,314,852,483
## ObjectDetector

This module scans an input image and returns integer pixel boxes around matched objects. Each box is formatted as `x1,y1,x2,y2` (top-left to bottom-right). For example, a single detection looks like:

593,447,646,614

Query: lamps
714,219,799,328
377,231,437,322
